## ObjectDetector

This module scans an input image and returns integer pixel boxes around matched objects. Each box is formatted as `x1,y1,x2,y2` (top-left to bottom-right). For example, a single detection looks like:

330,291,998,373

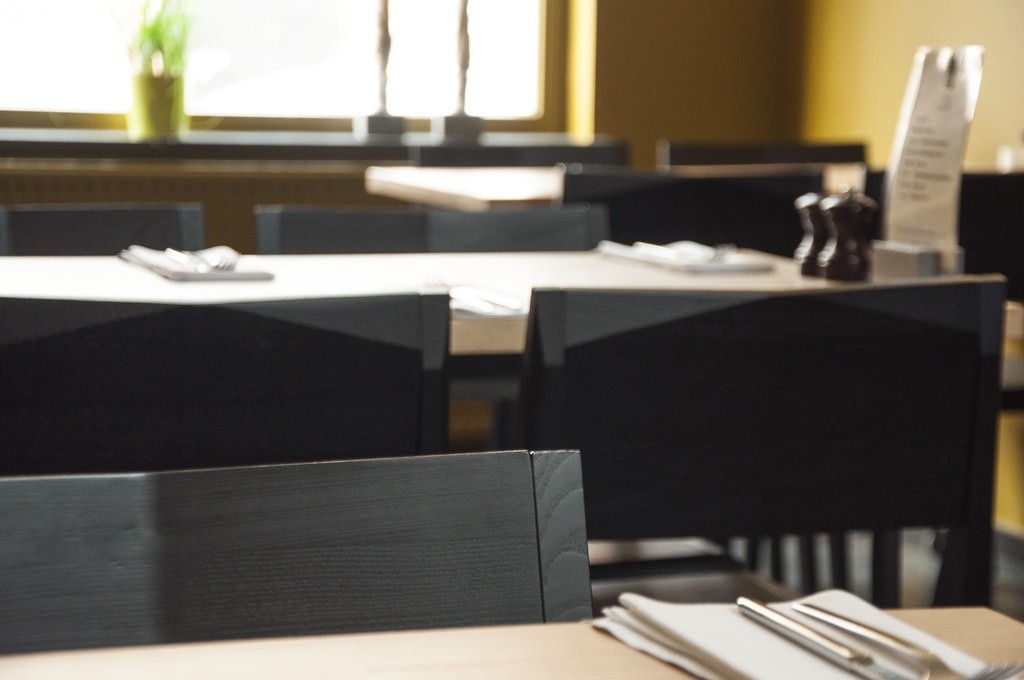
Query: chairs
657,136,873,166
514,268,1010,602
0,197,208,256
412,139,646,167
0,287,461,482
250,202,445,255
0,445,595,653
545,164,840,245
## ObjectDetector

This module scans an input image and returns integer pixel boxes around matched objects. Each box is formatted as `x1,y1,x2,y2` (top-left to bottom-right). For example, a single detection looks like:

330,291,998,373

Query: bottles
813,186,875,282
794,194,829,279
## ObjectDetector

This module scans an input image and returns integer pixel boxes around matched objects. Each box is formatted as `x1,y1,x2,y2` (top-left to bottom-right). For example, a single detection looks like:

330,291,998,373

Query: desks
0,236,901,354
366,165,864,217
0,603,1024,680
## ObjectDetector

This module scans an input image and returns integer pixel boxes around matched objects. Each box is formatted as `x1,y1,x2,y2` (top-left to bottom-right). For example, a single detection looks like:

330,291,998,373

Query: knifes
737,595,905,680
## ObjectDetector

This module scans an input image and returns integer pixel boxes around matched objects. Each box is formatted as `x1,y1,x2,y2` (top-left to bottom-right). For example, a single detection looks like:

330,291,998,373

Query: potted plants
122,0,199,139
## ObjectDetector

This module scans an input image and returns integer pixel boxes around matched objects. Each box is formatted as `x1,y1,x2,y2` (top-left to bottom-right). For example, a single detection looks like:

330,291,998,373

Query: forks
185,242,243,273
795,600,1023,680
